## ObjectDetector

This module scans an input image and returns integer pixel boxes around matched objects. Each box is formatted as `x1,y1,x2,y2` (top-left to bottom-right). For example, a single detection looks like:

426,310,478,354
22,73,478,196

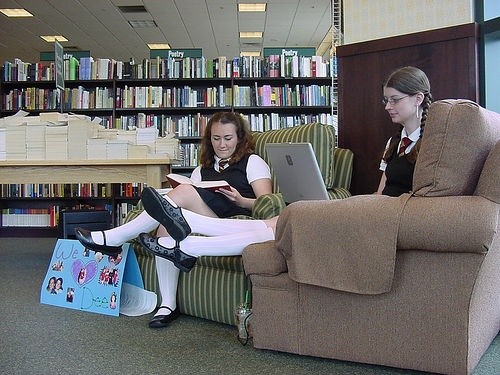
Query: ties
398,137,413,159
219,158,229,172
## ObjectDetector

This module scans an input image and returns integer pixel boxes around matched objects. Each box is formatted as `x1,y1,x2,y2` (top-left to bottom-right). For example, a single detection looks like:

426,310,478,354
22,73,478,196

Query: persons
138,66,432,274
73,112,273,329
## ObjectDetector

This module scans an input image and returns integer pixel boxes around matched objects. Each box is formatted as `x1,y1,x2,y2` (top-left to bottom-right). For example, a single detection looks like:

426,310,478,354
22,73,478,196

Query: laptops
265,142,331,204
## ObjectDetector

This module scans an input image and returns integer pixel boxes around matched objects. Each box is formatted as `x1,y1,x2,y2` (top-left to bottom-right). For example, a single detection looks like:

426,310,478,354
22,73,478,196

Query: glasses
381,96,408,105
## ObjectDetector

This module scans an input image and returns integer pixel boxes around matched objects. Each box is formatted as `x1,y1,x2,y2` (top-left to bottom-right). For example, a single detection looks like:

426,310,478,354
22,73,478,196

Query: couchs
240,99,500,375
120,123,354,328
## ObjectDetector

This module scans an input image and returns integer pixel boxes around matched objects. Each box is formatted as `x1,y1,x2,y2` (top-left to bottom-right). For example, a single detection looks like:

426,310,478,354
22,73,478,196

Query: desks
0,158,182,239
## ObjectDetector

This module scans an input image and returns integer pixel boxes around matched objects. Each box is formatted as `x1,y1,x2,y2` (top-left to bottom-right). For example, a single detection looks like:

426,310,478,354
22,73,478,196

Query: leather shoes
75,227,123,256
149,306,179,328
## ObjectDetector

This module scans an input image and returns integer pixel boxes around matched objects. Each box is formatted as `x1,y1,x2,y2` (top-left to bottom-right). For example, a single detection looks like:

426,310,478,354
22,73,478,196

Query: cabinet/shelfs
0,61,338,239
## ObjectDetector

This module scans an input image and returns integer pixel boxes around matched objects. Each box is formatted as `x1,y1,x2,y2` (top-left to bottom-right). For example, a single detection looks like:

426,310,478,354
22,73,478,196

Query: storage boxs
62,211,111,238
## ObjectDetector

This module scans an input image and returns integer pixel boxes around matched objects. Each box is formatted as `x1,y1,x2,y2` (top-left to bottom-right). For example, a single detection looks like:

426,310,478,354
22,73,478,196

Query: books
0,183,153,227
0,111,215,168
0,49,338,109
165,173,232,194
238,112,337,138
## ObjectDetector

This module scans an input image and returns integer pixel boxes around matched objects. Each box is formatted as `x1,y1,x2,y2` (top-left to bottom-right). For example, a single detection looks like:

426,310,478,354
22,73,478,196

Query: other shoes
141,187,191,242
138,232,197,274
